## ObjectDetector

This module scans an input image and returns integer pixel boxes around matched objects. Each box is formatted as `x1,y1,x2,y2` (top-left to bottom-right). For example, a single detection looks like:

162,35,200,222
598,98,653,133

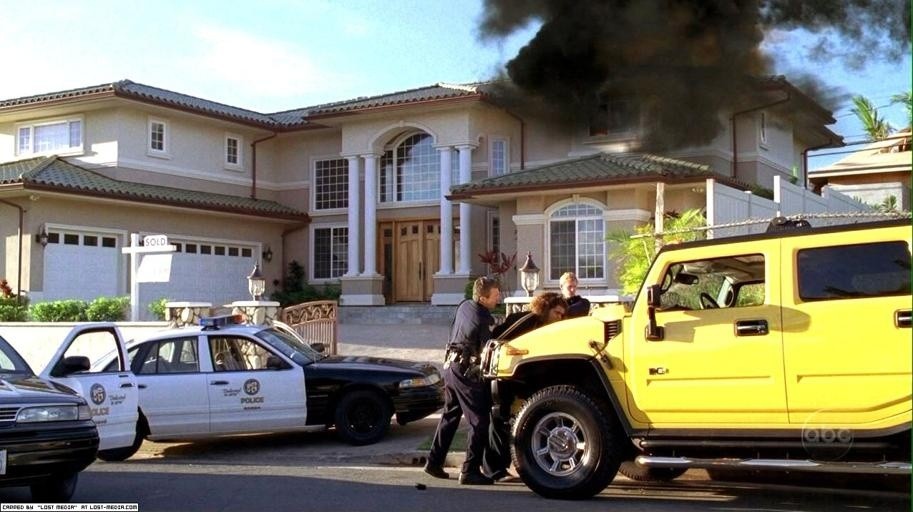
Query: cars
0,323,138,502
75,309,443,463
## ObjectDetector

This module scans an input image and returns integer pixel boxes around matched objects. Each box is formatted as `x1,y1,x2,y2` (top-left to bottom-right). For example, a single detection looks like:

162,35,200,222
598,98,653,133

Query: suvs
473,214,912,505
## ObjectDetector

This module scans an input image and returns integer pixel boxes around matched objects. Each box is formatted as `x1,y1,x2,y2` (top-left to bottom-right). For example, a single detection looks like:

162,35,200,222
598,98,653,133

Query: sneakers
424,456,449,479
458,470,493,485
496,473,516,482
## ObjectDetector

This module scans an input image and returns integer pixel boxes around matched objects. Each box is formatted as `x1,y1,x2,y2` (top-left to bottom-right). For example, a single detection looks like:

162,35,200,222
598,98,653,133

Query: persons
481,292,569,481
555,270,590,318
423,275,500,484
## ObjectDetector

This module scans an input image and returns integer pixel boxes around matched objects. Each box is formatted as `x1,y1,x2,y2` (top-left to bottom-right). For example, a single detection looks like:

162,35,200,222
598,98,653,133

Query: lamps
516,251,540,300
245,261,267,301
